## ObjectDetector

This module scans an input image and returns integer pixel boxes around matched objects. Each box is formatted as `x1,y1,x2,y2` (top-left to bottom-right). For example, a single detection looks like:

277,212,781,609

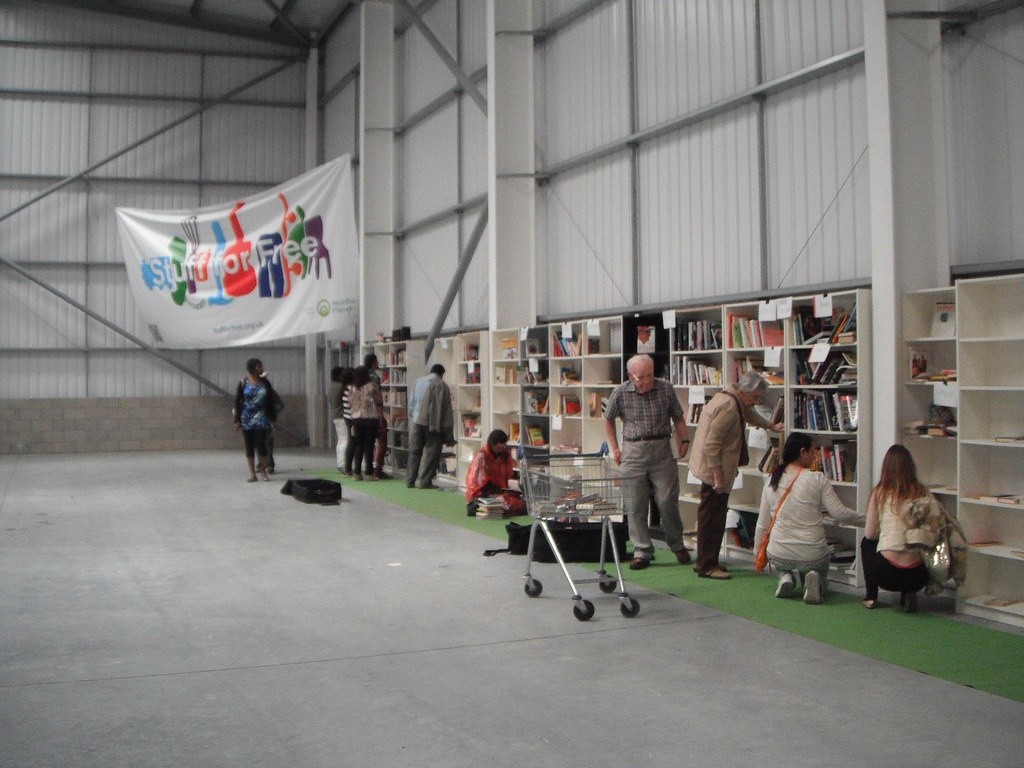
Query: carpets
310,466,1024,706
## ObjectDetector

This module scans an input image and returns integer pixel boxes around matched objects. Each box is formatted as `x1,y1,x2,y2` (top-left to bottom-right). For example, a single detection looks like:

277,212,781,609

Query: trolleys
518,441,640,621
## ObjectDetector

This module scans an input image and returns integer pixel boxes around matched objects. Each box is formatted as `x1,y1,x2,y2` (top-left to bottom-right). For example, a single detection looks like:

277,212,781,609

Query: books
589,319,722,424
503,479,523,493
383,451,391,466
379,349,406,406
386,431,408,449
438,452,456,473
557,493,620,515
756,438,857,481
382,407,407,430
462,390,481,439
727,304,857,431
476,497,508,518
464,344,480,384
494,331,581,445
903,300,1024,607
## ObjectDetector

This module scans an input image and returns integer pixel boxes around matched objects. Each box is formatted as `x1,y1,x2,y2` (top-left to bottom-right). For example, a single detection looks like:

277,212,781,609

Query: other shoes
904,593,917,612
256,465,261,472
676,548,690,563
862,597,877,608
775,574,793,597
406,484,414,488
267,467,274,473
693,563,726,572
697,567,731,579
337,466,393,482
803,571,819,604
419,484,439,489
629,557,648,569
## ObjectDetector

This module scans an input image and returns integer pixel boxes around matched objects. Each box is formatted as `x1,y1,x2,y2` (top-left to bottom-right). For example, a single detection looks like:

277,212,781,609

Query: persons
861,445,934,613
406,364,457,489
603,354,692,569
754,433,867,603
328,366,379,481
255,367,284,475
364,353,394,480
465,429,528,518
233,358,276,482
690,372,768,579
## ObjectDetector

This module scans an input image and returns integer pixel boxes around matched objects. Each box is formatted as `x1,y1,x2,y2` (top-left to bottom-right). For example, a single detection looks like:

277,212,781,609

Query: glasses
631,368,654,380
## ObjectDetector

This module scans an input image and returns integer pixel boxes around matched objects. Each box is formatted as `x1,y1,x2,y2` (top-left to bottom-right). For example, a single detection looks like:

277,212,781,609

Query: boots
246,456,257,482
258,455,270,482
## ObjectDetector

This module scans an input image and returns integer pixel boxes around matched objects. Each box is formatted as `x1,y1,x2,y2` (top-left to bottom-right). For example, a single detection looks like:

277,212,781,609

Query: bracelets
681,441,690,444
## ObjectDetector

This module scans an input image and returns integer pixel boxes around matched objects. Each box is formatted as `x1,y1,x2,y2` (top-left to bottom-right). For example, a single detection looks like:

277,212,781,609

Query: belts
623,435,668,441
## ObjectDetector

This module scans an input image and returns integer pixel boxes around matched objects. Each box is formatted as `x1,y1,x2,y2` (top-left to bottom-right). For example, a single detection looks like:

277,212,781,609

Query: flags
115,154,360,349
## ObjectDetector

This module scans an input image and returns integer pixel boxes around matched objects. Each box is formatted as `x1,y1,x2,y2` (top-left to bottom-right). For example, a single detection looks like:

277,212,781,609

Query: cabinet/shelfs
361,270,1024,637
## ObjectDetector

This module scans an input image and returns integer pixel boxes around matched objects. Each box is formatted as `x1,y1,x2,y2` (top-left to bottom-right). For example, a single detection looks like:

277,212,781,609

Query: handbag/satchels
755,533,769,574
738,436,749,466
921,523,952,582
